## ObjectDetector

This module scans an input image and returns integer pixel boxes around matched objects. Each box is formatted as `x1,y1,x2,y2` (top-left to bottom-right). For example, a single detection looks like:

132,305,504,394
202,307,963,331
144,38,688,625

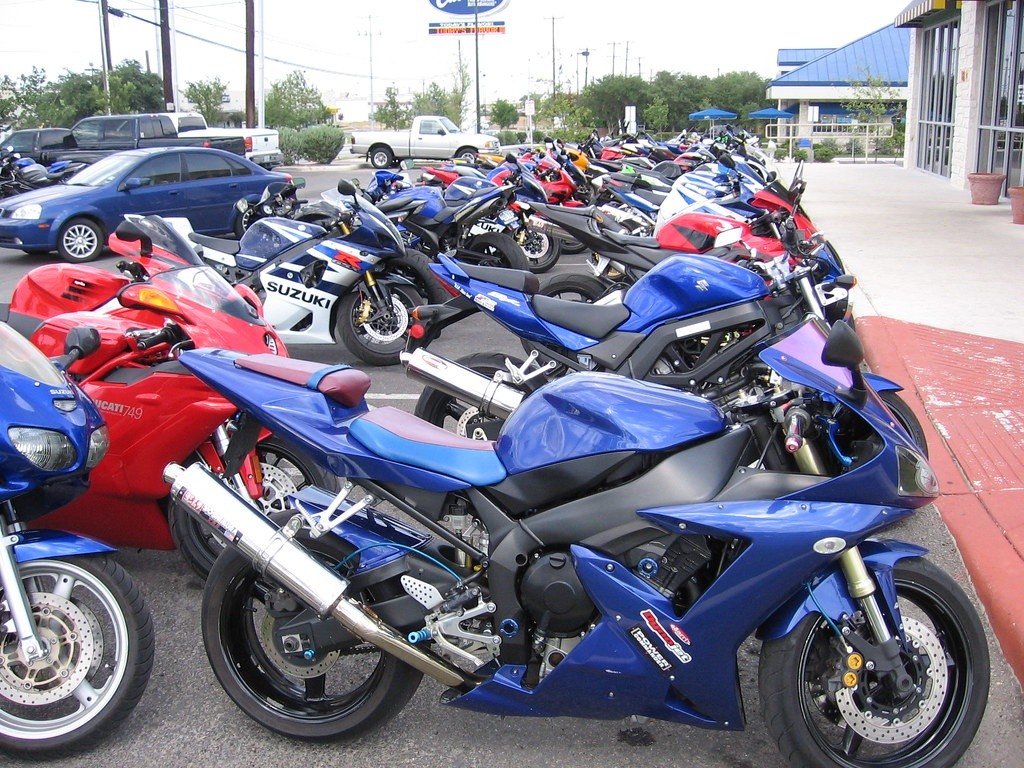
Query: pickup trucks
0,127,126,165
69,115,249,162
350,114,502,168
158,112,283,171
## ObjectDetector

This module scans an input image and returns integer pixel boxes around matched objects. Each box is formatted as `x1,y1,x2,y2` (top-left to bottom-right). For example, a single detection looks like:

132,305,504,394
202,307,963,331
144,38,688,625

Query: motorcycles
162,214,990,768
0,297,154,767
111,179,432,365
0,146,87,199
238,116,862,442
0,211,341,588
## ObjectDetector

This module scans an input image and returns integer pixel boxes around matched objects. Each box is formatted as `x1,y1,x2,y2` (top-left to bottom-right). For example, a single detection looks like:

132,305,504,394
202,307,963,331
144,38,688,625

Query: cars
0,145,302,263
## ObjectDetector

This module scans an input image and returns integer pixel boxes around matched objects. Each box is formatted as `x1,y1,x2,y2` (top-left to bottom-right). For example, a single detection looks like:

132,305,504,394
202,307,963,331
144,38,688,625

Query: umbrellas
689,109,738,139
748,108,794,141
